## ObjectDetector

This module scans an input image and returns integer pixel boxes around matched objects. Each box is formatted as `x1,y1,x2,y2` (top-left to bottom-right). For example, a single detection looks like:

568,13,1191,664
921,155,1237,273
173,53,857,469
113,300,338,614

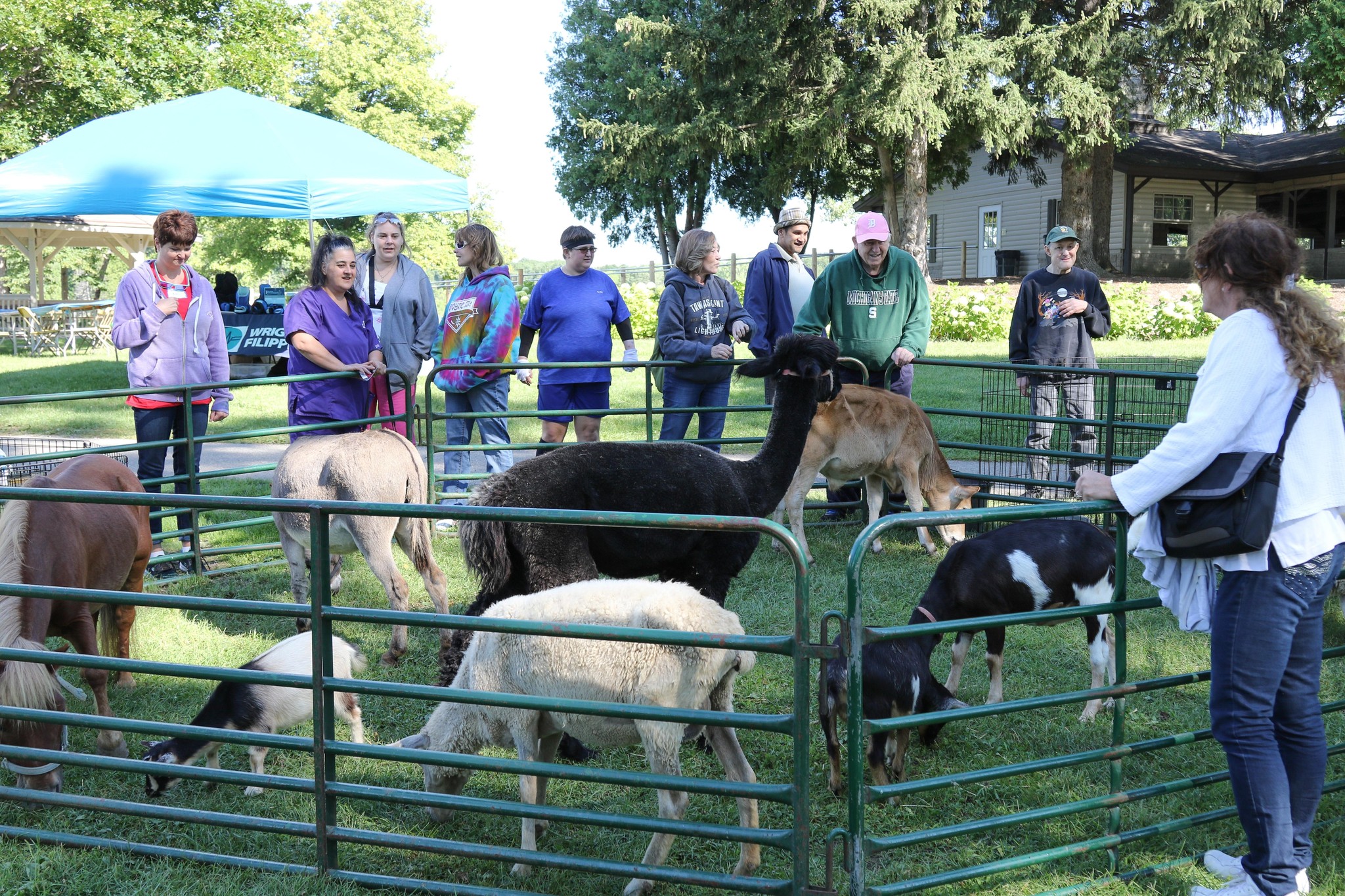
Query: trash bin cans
995,250,1021,277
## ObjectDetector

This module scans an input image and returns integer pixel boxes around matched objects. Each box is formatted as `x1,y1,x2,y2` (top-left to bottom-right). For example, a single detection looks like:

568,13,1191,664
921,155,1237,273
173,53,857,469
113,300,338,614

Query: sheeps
816,623,970,807
141,625,366,797
385,577,763,896
903,520,1118,723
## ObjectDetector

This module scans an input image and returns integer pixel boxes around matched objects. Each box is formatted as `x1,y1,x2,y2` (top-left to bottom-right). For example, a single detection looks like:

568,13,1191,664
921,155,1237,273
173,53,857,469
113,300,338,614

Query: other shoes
1020,490,1045,499
436,519,454,532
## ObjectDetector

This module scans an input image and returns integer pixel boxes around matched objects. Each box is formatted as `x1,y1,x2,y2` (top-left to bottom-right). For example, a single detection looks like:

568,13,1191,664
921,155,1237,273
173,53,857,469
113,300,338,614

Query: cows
769,382,981,567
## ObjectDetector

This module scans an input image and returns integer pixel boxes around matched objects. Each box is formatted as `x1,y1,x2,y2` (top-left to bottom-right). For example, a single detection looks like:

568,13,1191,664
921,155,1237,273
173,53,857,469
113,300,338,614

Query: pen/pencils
730,335,739,348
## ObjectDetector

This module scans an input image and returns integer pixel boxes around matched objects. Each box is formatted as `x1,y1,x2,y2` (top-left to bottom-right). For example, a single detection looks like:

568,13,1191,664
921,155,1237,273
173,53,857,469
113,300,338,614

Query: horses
0,455,155,791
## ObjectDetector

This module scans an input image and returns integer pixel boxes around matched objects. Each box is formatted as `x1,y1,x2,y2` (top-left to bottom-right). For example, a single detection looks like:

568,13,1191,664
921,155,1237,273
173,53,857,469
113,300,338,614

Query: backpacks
650,276,727,393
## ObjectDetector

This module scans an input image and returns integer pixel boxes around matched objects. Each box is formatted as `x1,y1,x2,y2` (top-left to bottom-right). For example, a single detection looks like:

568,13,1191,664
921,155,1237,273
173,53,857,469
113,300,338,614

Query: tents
0,86,472,260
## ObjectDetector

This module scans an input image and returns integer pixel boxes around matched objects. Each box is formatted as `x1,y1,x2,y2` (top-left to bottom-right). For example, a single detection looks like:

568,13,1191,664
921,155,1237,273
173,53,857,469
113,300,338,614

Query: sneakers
179,547,211,574
1203,848,1311,893
146,554,180,588
1191,875,1300,896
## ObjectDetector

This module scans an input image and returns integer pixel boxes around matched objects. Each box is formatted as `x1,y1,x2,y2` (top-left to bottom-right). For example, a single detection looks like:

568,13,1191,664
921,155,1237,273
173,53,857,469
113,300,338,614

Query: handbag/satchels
1158,377,1314,557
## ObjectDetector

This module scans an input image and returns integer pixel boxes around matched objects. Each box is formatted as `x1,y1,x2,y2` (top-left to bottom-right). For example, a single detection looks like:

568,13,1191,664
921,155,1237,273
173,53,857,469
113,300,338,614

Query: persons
1073,211,1345,896
111,208,233,581
353,212,439,450
1009,225,1111,500
655,230,758,454
791,210,931,520
431,223,520,531
516,226,639,457
283,231,387,445
742,206,829,359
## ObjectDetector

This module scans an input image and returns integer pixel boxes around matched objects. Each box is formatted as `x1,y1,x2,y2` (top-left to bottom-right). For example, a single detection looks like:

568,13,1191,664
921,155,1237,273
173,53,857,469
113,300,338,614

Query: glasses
154,260,190,291
456,240,468,249
572,248,597,254
375,218,402,225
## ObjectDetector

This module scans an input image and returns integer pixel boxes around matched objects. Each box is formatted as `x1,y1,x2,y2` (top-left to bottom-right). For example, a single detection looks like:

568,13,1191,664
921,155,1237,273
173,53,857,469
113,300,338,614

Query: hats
774,208,812,235
855,210,891,244
1046,225,1082,245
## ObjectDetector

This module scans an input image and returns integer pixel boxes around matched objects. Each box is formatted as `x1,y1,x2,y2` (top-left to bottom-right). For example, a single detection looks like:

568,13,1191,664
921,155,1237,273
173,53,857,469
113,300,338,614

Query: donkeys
273,424,456,663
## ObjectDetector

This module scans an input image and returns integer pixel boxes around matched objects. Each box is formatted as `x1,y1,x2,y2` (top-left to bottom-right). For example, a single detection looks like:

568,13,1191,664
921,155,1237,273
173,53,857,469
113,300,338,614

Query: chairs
0,305,118,356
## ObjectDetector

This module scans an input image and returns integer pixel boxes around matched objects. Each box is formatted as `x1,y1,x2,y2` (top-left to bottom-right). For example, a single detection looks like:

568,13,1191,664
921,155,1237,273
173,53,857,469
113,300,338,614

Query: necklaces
374,257,397,281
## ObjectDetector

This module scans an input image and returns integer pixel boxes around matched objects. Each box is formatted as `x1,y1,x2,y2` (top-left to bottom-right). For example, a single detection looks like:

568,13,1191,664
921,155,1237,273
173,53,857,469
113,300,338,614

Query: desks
0,300,116,354
221,310,290,366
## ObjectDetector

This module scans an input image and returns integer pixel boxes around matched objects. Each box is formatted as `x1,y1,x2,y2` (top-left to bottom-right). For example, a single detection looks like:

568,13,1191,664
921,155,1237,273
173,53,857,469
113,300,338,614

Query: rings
1074,491,1083,499
